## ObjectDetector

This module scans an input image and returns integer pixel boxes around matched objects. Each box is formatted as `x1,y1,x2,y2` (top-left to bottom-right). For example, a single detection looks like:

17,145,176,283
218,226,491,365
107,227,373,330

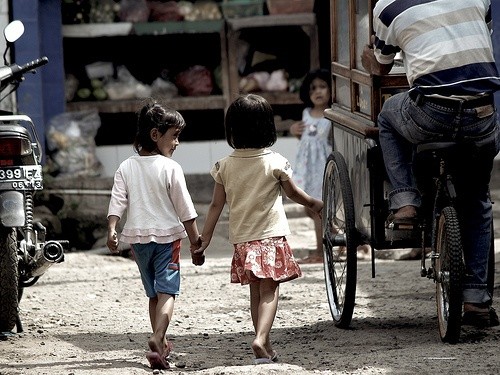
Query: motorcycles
0,18,71,338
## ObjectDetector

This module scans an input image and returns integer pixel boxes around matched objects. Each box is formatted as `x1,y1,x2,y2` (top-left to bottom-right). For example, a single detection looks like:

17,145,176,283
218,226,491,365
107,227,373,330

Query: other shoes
297,256,323,264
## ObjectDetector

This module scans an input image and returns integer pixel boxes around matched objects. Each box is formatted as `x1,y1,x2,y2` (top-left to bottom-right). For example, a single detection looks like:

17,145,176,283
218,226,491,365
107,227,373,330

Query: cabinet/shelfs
62,14,318,122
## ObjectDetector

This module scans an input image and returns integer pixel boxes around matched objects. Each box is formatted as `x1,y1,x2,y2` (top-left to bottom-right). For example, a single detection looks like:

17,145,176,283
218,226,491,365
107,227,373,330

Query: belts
409,92,495,110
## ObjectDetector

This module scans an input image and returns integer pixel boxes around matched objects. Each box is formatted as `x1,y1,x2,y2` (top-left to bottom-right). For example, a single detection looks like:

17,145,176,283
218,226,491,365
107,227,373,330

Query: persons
289,69,345,255
361,0,500,327
106,96,206,371
188,93,324,364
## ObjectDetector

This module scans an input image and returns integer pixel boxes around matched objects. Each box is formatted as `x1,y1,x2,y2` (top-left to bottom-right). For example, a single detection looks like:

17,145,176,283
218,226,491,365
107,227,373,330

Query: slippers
146,341,173,369
462,307,499,329
255,350,277,365
386,213,417,228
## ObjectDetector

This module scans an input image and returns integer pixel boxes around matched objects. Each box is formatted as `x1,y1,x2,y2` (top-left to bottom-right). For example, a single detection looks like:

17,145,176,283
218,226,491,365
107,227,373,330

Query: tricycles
317,0,470,348
